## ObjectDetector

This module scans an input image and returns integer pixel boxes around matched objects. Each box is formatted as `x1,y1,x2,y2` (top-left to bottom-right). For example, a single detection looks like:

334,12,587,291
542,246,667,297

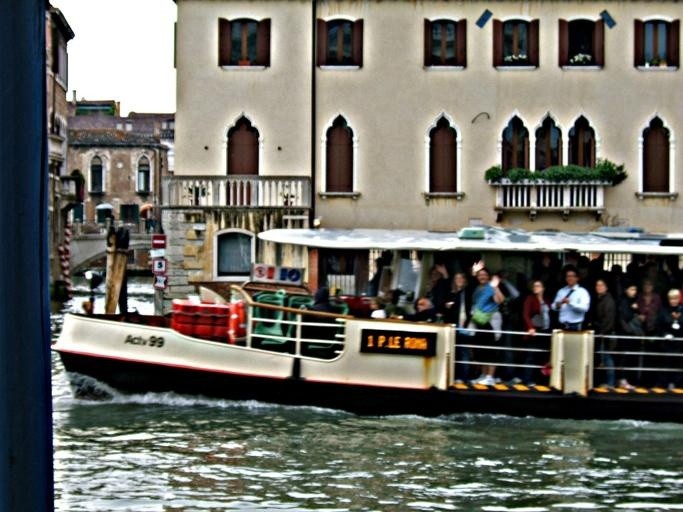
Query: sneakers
469,375,486,385
477,374,496,386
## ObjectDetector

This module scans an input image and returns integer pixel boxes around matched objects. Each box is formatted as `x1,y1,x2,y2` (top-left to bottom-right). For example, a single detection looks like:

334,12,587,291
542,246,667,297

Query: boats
55,221,683,421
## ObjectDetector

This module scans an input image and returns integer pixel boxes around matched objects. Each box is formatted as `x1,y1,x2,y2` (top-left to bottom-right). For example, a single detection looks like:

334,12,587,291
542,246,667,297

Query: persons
57,169,84,229
146,207,158,234
103,209,119,246
334,250,683,390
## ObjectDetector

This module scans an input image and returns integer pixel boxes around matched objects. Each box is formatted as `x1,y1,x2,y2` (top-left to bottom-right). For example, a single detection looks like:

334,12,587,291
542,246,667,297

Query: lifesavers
228,301,245,343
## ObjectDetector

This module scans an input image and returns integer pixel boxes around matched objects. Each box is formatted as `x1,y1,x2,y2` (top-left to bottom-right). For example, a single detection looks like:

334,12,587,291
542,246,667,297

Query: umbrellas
136,203,154,216
93,203,114,210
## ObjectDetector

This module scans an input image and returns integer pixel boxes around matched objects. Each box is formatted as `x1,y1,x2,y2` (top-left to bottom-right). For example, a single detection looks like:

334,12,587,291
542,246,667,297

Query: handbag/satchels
473,307,496,325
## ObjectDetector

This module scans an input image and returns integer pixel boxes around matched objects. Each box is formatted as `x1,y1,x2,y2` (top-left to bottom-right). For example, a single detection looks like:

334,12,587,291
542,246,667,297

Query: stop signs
151,234,166,249
